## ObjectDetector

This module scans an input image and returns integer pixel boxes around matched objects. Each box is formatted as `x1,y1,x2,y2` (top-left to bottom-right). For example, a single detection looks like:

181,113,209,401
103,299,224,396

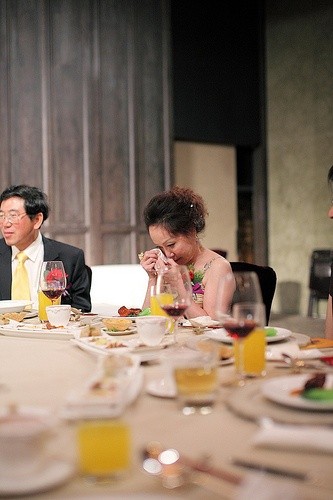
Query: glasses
0,211,26,223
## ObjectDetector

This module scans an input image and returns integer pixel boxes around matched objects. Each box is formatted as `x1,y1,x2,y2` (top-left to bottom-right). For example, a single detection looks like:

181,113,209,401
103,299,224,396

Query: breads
0,312,26,325
101,319,132,331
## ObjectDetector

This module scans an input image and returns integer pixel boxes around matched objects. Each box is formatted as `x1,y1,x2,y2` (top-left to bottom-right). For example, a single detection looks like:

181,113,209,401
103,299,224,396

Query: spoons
281,352,327,369
188,318,223,329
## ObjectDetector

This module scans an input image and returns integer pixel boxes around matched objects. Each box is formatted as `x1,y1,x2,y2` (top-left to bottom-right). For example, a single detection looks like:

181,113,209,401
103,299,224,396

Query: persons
138,185,237,321
323,163,333,340
0,182,93,315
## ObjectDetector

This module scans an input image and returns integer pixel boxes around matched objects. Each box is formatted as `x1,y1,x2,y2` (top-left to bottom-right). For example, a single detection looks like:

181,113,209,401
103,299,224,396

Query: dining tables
0,308,332,499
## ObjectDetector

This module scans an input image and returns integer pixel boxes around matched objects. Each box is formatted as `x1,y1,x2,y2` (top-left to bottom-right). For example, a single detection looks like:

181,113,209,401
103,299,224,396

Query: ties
12,251,31,300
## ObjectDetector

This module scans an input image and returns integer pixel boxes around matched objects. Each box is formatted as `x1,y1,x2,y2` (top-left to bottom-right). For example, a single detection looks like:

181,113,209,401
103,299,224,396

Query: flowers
43,266,71,298
181,264,206,294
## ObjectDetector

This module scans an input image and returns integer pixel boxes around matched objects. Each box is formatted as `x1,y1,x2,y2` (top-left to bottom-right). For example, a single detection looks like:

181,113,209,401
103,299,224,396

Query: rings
138,252,144,261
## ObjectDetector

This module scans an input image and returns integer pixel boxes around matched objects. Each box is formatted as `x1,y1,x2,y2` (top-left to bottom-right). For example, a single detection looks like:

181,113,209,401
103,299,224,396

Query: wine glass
216,270,264,389
39,261,66,305
156,265,192,348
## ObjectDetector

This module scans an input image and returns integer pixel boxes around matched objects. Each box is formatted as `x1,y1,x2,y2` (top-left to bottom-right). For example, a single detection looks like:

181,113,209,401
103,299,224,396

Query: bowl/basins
0,300,35,313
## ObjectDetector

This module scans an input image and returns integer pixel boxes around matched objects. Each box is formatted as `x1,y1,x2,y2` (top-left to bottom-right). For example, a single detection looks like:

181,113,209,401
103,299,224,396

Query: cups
151,284,175,335
231,302,265,379
37,280,61,324
134,316,172,347
75,415,132,481
45,304,72,328
172,342,224,417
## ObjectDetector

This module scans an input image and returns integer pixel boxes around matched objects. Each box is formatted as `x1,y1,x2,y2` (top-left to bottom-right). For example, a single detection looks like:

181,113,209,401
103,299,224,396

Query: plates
0,428,78,499
0,313,333,419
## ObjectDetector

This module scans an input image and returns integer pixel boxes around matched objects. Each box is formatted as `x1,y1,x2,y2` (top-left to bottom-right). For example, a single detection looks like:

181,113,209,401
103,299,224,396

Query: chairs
229,260,276,326
